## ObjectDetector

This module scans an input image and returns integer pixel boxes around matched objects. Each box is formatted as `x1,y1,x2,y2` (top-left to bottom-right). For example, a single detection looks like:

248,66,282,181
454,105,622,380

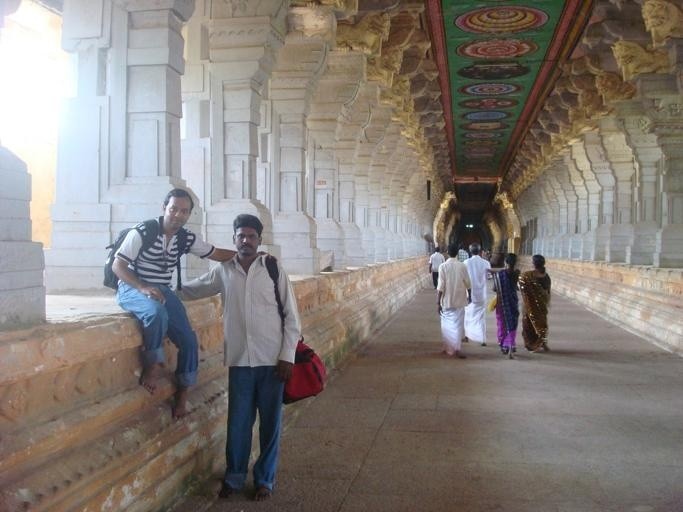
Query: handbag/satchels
280,338,334,406
487,295,499,312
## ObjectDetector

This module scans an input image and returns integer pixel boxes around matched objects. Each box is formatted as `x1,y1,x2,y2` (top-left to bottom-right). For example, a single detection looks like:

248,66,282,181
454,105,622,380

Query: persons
101,188,270,421
481,245,492,280
171,214,301,502
442,247,450,260
458,242,468,262
487,253,519,359
434,242,472,359
518,254,551,352
461,244,487,347
428,247,445,289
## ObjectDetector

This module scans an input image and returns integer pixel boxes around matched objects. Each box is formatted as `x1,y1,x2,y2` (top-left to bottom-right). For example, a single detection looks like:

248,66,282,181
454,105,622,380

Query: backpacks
102,226,137,293
